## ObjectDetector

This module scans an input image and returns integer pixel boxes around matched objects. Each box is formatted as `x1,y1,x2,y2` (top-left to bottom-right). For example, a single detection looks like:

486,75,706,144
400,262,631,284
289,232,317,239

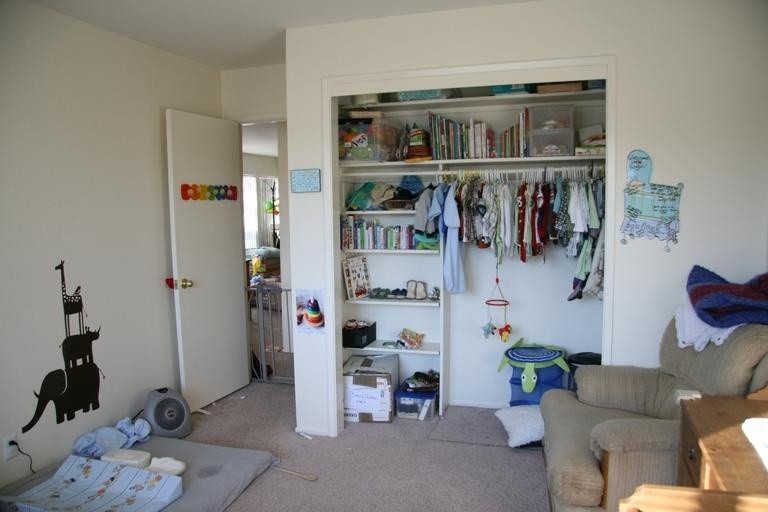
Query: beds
244,247,280,314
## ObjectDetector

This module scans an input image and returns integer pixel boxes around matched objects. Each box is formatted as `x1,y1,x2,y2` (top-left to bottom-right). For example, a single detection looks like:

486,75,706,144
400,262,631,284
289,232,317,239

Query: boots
407,279,427,300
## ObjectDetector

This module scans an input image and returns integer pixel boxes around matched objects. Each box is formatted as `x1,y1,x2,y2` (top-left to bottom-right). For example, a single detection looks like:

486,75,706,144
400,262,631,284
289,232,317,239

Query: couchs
540,312,767,511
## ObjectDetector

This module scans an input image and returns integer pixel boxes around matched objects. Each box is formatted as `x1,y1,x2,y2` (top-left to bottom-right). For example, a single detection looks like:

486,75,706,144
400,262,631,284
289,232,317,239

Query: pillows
495,402,544,450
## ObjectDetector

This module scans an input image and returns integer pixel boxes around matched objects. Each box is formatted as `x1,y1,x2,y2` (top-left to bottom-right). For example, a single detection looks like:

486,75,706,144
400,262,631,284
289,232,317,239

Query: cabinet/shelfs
334,79,606,169
339,162,448,424
615,394,767,512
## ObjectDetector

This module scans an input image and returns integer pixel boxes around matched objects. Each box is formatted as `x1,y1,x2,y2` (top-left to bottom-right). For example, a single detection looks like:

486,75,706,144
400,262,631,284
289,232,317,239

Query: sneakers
370,287,407,299
345,317,368,330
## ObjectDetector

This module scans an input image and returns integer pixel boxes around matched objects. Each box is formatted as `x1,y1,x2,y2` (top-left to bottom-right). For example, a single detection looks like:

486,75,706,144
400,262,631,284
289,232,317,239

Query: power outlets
3,431,20,461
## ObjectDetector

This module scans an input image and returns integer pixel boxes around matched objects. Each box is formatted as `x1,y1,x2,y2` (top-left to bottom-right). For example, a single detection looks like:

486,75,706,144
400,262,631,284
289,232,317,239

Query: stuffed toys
482,276,511,343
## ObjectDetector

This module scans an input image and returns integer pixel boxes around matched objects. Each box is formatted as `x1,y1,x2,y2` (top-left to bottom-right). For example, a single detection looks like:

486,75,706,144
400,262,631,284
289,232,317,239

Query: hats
371,181,397,204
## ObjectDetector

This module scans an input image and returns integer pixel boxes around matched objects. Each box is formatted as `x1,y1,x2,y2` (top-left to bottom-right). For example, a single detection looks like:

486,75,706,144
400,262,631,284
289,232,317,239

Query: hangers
433,165,603,186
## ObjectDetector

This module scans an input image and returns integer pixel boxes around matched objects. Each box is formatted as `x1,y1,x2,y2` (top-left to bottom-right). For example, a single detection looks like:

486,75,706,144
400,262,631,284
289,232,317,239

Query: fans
145,387,192,438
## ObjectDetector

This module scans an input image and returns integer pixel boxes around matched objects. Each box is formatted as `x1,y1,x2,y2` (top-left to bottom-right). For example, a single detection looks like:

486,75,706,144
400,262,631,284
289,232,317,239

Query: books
341,261,354,302
349,256,371,300
339,214,415,252
426,105,529,159
